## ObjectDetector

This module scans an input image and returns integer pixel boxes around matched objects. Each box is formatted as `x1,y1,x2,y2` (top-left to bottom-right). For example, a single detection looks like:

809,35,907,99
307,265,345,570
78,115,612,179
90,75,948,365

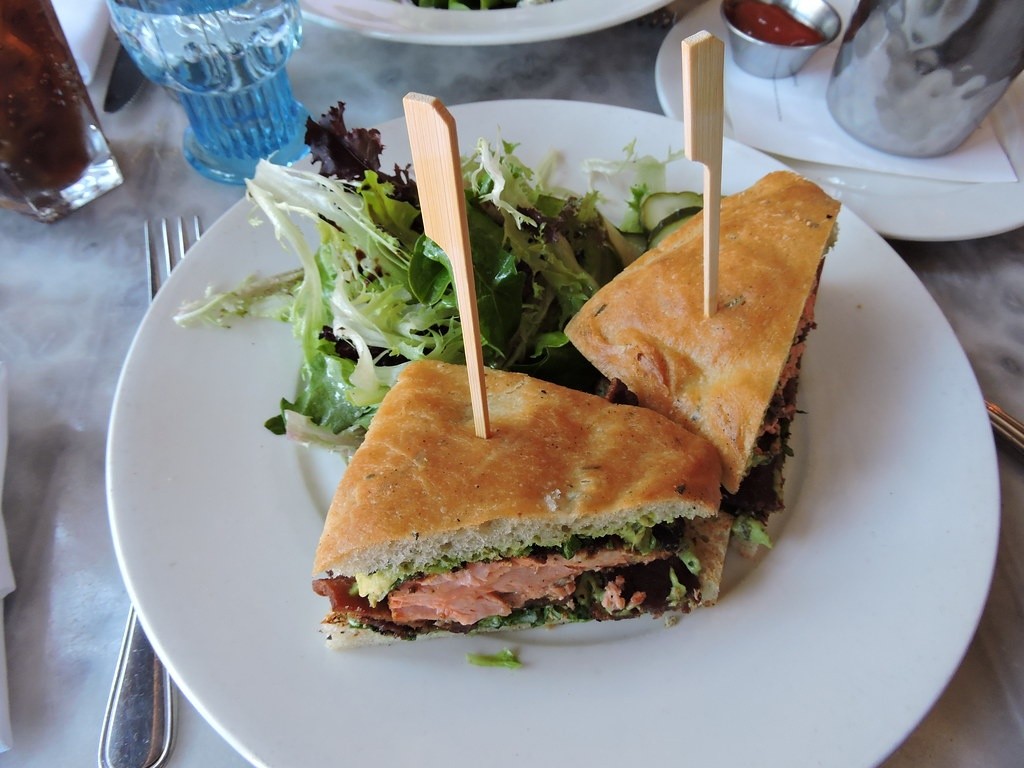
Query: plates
285,0,674,46
105,97,997,768
654,0,1024,243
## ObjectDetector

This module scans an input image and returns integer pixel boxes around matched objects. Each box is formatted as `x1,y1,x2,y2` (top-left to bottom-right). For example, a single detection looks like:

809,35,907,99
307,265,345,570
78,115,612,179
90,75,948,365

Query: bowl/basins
719,0,840,79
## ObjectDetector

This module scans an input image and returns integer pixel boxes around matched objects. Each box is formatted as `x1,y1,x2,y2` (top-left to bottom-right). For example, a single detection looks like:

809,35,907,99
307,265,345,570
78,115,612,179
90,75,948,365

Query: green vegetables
172,100,696,446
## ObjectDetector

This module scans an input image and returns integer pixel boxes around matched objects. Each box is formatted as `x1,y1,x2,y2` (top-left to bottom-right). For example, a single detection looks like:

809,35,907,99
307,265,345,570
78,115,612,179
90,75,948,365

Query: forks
90,216,207,768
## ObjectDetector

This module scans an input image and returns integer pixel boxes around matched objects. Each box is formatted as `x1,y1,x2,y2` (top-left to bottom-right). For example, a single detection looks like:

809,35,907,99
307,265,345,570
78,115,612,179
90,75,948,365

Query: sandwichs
310,360,733,649
562,171,842,561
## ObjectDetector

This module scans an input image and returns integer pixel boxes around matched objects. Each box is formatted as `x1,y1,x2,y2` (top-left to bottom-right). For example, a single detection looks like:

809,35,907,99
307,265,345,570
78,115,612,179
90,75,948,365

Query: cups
0,1,126,222
828,0,1024,158
107,0,315,184
